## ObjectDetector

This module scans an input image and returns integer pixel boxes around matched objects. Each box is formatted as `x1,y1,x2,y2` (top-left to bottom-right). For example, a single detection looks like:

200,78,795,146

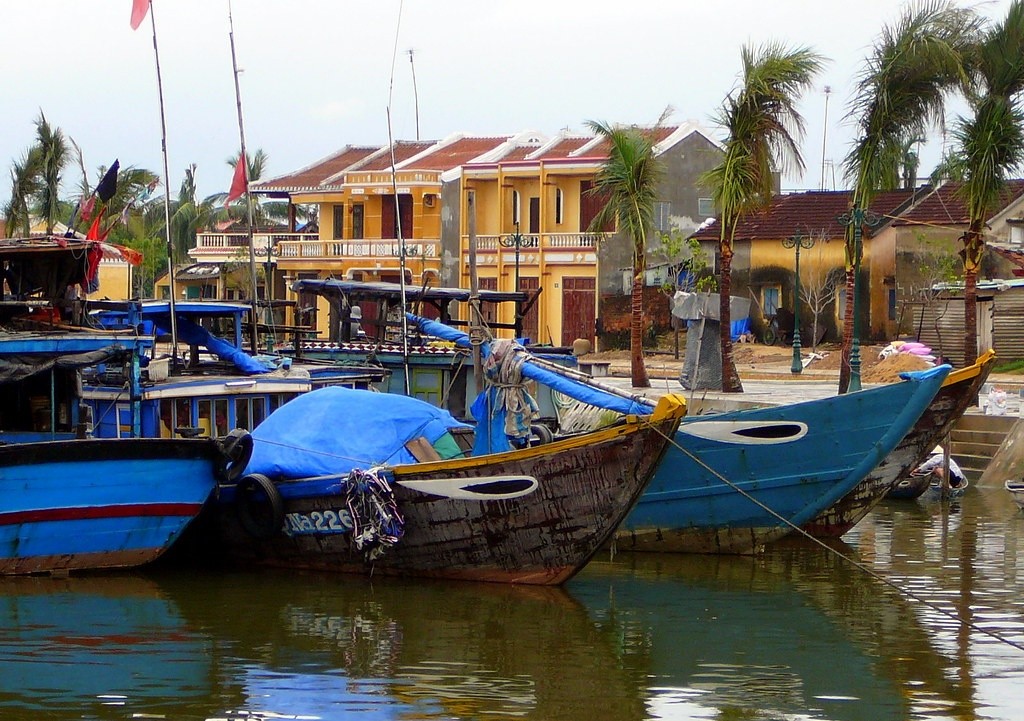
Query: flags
96,160,120,203
86,217,99,261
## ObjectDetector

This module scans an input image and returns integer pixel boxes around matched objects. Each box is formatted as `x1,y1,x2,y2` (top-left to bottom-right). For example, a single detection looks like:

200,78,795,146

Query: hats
930,444,944,454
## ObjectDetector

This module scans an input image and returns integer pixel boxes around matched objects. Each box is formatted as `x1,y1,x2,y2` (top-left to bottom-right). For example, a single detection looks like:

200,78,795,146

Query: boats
266,280,580,435
783,349,997,542
282,361,393,395
215,394,689,588
884,468,934,500
1004,480,1024,511
80,299,309,448
602,363,954,557
0,331,241,578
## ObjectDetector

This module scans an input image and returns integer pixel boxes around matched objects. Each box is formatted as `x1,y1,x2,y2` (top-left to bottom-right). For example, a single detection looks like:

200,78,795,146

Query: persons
912,445,964,490
339,305,362,337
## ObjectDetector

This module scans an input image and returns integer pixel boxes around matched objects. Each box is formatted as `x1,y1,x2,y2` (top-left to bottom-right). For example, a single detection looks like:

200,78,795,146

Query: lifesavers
232,472,287,539
212,425,255,485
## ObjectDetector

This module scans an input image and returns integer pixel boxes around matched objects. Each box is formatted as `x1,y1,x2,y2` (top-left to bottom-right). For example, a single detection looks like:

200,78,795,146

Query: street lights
390,237,420,286
783,223,816,372
836,199,878,393
498,222,537,338
